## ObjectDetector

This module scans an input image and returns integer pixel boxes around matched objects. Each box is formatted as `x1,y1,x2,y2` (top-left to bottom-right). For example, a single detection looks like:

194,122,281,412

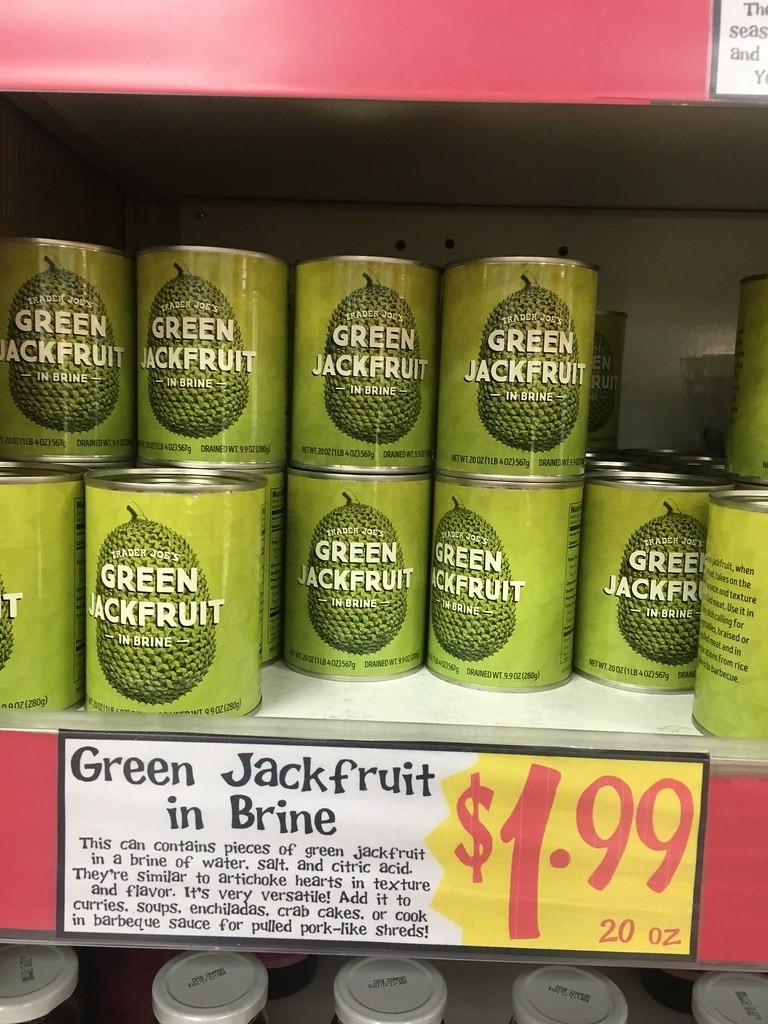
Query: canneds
0,237,768,743
0,943,768,1024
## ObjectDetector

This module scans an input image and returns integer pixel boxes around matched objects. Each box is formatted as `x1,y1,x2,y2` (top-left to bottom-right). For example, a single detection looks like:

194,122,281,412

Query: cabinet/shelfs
0,0,768,976
